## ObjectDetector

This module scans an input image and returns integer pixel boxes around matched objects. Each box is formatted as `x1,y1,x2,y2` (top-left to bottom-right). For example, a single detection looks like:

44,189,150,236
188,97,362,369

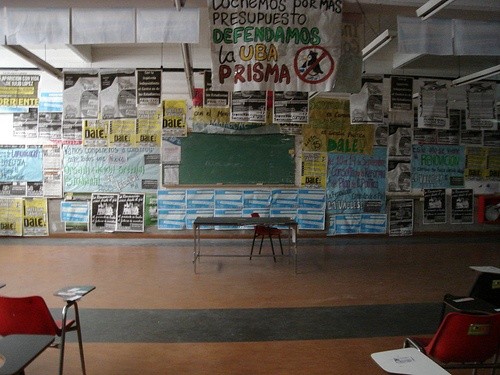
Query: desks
191,216,299,275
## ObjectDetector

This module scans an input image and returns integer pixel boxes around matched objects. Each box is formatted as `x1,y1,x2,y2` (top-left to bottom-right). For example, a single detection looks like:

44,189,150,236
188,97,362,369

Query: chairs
250,212,285,263
0,281,96,375
372,309,500,375
440,265,500,326
